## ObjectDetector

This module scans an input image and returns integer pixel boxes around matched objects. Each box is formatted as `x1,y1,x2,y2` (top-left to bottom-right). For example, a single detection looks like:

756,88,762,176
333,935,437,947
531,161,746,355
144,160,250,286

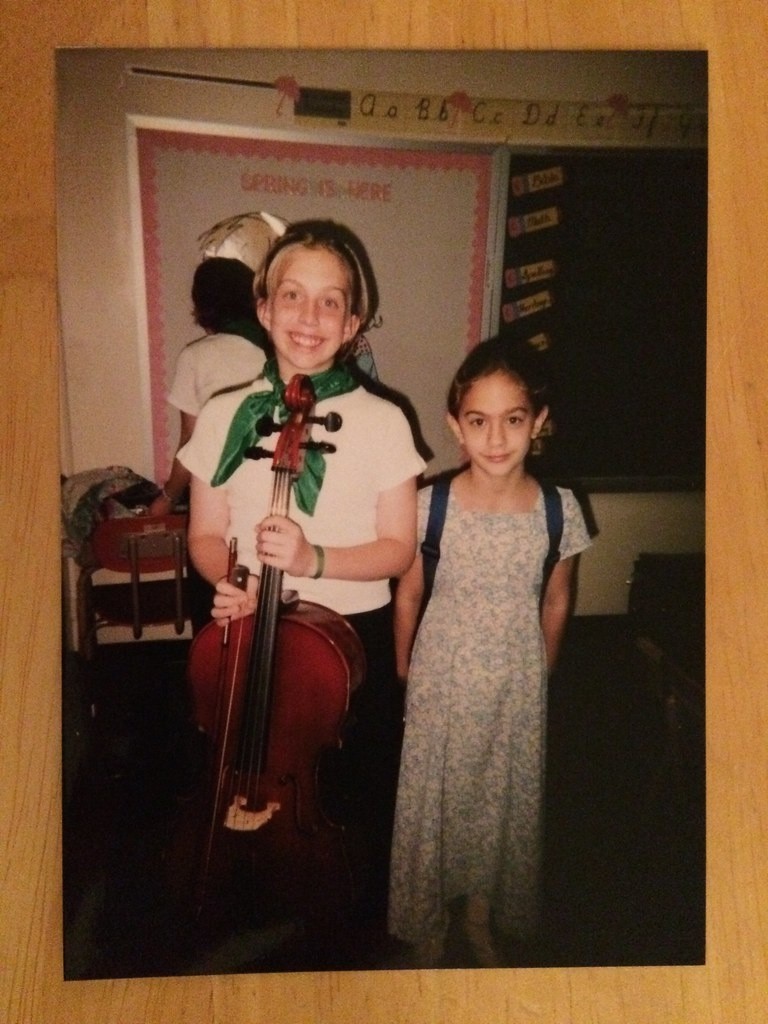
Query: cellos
174,372,369,953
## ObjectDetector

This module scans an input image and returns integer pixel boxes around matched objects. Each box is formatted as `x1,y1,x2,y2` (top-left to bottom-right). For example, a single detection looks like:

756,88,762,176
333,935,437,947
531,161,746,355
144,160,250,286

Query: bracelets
162,488,173,503
313,544,324,579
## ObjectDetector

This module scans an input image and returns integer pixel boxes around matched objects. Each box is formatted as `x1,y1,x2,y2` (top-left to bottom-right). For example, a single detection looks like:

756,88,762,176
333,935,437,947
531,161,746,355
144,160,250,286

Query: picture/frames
1,0,766,1024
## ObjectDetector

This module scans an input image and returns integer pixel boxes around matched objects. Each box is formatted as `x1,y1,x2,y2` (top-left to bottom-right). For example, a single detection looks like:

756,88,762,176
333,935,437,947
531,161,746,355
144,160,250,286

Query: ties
209,355,363,518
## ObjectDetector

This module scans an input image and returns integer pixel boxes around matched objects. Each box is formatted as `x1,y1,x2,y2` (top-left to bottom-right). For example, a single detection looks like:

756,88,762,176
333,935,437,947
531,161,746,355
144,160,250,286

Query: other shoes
403,949,431,968
461,935,508,968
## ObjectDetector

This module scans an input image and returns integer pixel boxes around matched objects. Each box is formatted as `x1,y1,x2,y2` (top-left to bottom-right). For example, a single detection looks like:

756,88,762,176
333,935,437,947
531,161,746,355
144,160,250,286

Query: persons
178,232,426,958
146,258,274,645
392,336,594,969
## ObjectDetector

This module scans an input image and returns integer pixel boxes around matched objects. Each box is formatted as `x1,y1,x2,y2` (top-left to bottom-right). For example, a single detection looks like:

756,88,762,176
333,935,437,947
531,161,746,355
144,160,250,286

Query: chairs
79,512,194,721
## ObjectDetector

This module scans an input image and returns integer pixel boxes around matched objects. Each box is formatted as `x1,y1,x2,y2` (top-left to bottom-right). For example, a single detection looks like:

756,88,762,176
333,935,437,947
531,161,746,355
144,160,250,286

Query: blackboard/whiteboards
478,145,708,496
